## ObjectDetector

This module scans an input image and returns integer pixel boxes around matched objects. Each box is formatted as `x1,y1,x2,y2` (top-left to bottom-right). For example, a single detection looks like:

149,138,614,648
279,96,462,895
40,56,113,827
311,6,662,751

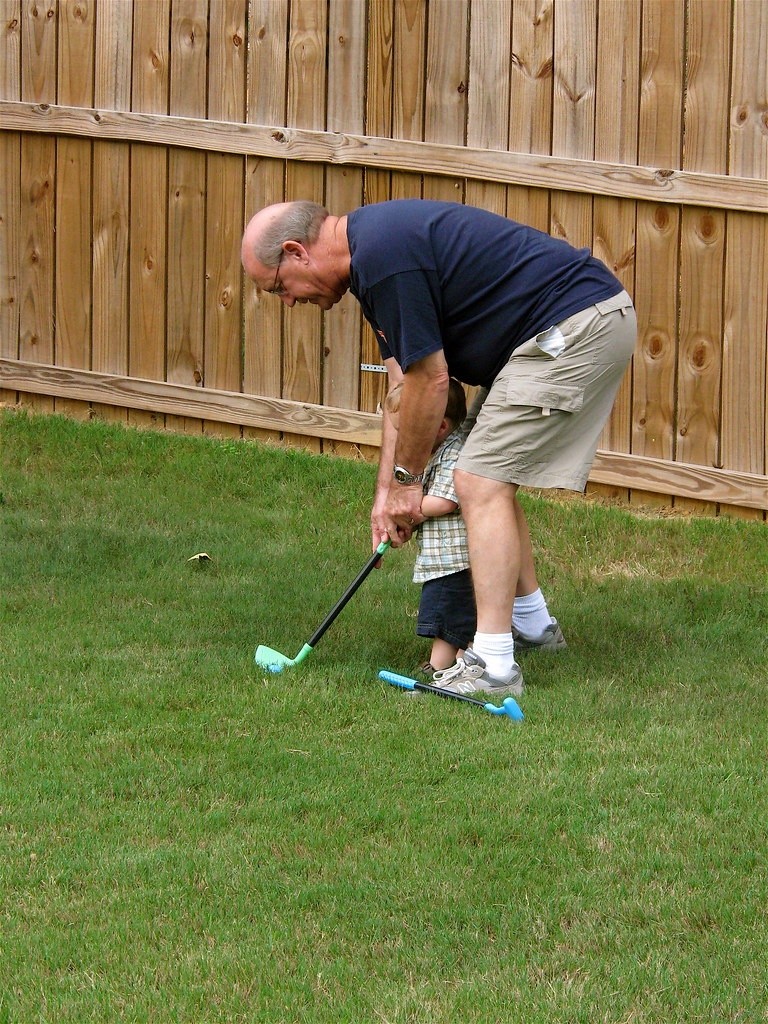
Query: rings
409,519,414,524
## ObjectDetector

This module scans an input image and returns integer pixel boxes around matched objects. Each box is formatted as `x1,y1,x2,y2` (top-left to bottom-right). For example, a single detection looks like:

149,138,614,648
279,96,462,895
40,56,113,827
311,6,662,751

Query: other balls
264,664,281,676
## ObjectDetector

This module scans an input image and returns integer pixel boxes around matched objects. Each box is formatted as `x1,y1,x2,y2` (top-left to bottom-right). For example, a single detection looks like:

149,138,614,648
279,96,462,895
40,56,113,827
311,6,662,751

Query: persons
386,377,477,676
241,201,636,698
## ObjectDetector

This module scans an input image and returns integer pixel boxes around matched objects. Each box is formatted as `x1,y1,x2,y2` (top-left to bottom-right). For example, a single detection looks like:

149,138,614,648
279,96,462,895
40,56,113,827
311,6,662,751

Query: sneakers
409,660,457,681
513,616,567,654
401,647,523,697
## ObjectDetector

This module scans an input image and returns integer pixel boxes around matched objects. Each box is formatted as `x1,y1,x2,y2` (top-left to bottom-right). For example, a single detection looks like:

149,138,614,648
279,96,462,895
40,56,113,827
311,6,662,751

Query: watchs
391,465,425,484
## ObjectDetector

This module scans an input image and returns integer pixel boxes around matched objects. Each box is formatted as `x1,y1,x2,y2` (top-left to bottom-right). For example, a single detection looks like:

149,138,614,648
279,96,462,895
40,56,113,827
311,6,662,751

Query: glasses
269,240,302,296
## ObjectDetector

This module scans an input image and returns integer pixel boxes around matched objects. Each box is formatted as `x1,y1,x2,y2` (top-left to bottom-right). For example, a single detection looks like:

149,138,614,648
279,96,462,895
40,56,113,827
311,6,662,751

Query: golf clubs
379,671,526,721
254,524,401,672
